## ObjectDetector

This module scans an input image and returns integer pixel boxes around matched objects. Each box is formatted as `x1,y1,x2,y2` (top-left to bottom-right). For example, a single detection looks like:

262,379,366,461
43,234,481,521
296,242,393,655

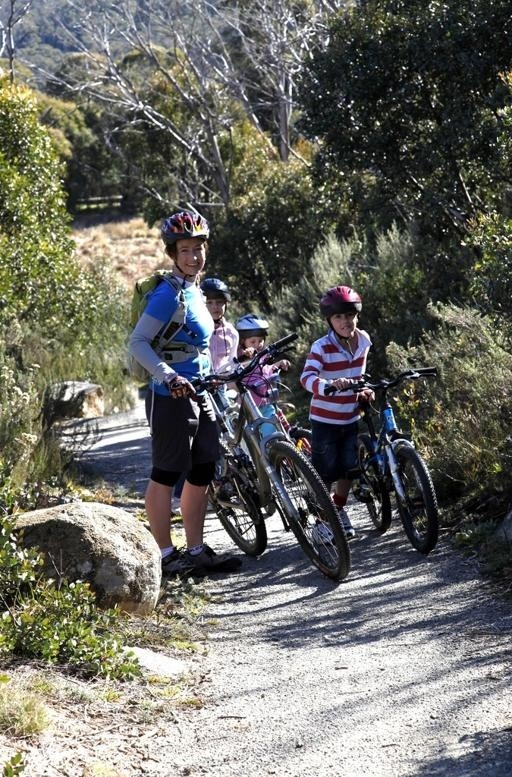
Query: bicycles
318,366,444,555
237,368,312,518
159,327,352,584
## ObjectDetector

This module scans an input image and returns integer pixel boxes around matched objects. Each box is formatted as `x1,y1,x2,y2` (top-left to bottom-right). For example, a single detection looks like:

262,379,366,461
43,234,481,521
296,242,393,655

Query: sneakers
310,519,333,545
161,548,205,577
185,544,243,573
329,509,355,539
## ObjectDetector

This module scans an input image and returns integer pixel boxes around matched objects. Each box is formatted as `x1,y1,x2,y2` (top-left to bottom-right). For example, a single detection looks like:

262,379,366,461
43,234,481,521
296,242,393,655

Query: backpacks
126,268,188,387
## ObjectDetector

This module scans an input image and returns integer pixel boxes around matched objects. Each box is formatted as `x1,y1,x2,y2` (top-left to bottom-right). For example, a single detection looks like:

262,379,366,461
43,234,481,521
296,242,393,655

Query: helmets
320,285,362,317
200,278,230,297
234,313,268,339
161,210,212,248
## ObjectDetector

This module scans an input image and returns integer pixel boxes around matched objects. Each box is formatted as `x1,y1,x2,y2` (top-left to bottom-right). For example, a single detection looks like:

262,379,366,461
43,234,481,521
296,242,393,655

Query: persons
232,313,290,452
170,276,239,515
127,211,242,582
299,286,375,547
